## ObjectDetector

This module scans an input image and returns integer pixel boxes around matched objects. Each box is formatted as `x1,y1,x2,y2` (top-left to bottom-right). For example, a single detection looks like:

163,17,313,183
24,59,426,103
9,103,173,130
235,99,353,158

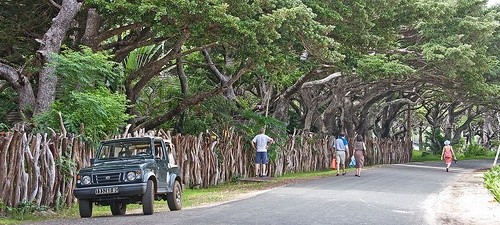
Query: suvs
71,136,183,218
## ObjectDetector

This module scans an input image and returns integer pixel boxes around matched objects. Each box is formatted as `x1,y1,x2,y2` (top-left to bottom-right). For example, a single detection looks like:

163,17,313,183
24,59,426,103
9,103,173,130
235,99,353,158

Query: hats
444,140,450,145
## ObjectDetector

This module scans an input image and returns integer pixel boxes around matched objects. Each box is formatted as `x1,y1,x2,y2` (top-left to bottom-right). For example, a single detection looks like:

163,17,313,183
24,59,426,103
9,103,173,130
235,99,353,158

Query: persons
440,140,457,172
352,135,367,177
332,131,350,176
251,127,273,176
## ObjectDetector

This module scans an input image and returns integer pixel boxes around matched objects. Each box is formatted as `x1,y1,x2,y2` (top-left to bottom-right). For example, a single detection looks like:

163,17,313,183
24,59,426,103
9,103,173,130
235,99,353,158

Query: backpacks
444,146,451,158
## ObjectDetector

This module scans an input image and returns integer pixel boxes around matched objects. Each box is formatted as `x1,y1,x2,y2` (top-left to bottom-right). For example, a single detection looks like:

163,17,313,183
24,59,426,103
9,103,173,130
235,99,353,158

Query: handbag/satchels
349,158,356,167
331,156,336,169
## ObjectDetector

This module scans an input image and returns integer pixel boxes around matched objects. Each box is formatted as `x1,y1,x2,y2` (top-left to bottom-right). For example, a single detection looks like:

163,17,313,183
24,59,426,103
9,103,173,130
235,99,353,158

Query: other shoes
336,172,360,177
446,168,448,172
